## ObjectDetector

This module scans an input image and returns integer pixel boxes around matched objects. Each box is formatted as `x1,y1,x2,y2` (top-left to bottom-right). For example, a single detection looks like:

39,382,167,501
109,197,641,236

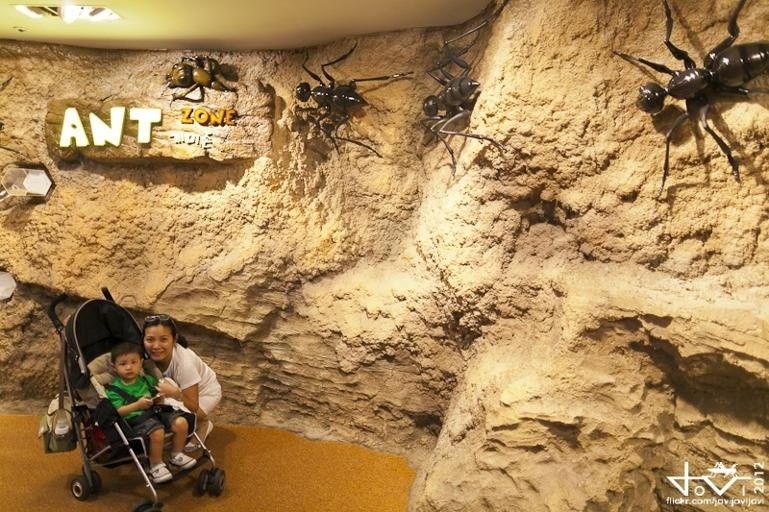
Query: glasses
144,314,177,329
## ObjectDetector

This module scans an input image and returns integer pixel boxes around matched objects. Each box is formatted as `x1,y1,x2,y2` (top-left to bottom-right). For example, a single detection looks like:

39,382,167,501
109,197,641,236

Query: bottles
55,409,70,436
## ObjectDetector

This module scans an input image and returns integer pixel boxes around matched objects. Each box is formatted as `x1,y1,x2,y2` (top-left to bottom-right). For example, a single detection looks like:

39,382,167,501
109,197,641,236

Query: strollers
44,284,227,512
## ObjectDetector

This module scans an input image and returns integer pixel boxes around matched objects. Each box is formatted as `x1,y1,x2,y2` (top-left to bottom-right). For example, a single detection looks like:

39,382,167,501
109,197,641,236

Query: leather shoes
184,419,214,453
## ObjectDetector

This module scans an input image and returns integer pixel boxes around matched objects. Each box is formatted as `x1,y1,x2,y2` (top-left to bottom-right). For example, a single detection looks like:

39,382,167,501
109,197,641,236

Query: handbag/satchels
37,393,77,453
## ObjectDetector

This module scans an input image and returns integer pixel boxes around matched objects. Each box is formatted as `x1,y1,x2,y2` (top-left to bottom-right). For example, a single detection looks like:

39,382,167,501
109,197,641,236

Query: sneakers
149,461,173,483
167,452,197,471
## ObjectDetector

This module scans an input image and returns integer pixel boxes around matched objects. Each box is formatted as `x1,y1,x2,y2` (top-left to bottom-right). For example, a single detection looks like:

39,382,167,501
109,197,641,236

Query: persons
141,312,224,453
108,339,197,484
